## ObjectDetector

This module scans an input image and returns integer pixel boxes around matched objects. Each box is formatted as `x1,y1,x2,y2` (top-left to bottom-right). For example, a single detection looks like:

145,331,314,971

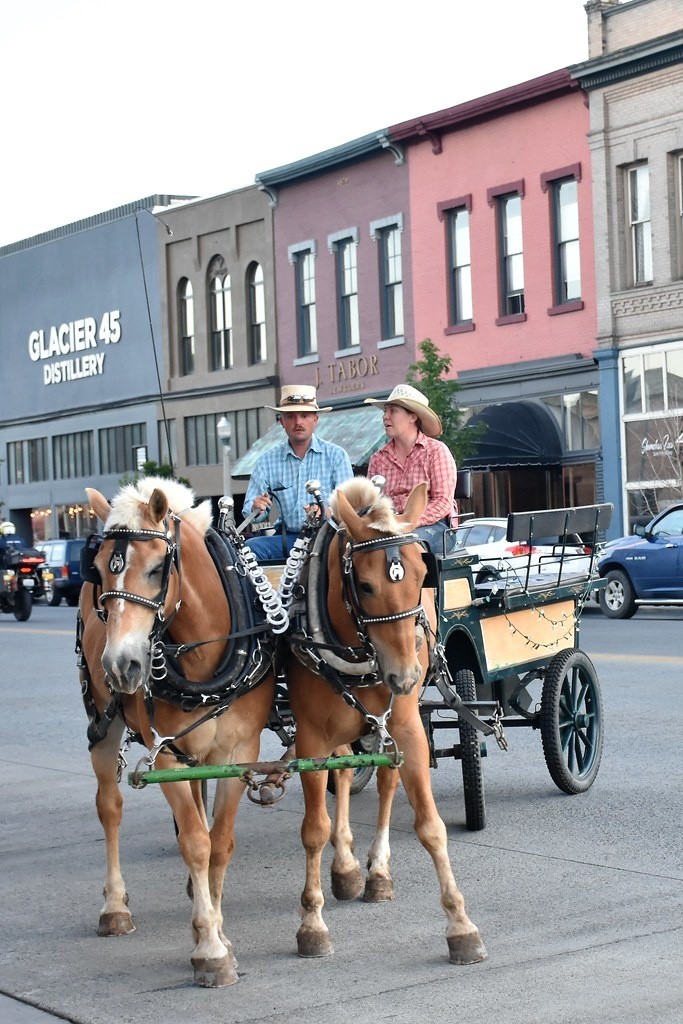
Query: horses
76,474,489,991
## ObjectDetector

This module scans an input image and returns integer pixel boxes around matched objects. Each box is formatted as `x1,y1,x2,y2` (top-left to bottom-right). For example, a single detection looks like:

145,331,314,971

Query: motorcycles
0,547,55,622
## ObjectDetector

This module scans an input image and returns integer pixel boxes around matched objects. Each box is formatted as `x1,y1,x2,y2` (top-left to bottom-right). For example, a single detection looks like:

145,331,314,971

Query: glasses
280,395,318,404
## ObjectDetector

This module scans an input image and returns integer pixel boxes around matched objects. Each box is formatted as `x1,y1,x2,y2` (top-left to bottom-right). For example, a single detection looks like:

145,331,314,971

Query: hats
365,384,443,441
263,383,335,421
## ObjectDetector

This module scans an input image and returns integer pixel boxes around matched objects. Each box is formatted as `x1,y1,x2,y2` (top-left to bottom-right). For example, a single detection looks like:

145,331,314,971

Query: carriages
75,474,607,989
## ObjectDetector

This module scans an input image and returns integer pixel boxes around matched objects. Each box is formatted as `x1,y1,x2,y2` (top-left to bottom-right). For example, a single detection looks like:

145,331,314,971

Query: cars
449,517,597,602
592,502,683,620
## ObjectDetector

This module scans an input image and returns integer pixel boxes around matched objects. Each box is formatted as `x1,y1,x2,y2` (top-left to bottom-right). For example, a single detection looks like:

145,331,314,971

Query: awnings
450,399,561,470
223,406,394,480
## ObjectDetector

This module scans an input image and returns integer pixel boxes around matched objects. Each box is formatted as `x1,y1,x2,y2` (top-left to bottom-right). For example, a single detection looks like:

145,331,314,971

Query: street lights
214,414,237,526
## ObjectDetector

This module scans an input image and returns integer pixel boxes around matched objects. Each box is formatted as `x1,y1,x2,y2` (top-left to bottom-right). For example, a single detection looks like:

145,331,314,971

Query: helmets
0,521,16,536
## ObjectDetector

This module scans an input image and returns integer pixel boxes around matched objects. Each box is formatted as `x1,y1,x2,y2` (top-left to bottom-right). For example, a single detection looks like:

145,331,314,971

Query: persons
364,384,458,553
242,385,354,560
0,521,28,571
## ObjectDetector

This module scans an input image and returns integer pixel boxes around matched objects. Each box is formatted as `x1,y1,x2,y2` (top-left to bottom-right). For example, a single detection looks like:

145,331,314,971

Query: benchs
474,502,615,597
433,511,480,571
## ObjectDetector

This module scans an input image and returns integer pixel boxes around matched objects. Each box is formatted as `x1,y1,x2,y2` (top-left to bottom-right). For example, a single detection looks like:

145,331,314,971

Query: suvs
33,537,99,607
